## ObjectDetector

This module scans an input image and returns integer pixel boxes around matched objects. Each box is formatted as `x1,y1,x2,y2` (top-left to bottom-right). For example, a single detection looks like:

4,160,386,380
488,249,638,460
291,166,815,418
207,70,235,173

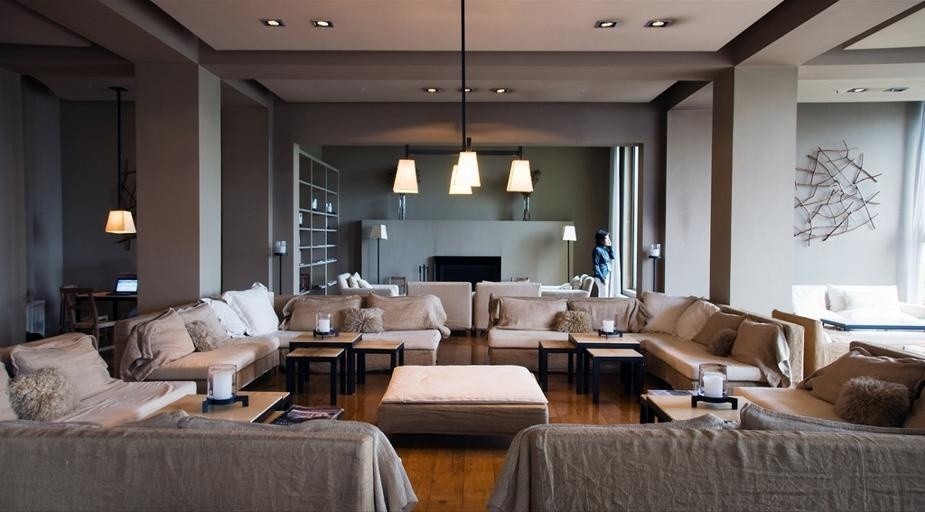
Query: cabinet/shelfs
273,142,340,296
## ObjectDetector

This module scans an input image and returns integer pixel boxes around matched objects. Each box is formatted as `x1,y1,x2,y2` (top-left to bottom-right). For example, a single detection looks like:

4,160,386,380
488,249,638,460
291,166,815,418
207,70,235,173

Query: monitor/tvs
432,256,502,292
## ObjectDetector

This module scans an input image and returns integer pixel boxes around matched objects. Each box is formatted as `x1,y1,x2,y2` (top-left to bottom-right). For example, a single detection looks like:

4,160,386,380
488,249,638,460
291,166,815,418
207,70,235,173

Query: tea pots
207,364,237,401
316,313,334,332
700,363,729,398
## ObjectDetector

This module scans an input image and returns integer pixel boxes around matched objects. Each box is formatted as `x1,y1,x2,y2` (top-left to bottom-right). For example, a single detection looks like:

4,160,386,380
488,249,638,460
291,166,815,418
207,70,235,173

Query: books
269,402,342,427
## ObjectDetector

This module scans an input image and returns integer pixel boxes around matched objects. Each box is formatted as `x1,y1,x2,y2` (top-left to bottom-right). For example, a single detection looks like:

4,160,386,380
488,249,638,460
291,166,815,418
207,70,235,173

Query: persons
591,229,616,298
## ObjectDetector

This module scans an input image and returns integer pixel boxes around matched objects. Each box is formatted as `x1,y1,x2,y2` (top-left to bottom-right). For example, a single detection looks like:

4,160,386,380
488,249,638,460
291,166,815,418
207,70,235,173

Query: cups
603,321,615,332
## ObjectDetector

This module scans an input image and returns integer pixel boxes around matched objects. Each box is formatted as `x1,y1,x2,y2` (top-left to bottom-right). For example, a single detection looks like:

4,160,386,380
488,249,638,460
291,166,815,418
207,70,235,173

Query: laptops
106,277,138,295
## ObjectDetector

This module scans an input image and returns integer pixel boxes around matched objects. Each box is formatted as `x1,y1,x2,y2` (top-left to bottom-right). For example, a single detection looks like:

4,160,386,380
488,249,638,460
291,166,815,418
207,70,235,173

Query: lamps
103,210,137,235
368,224,388,284
562,225,578,283
393,0,533,196
273,240,288,295
648,247,662,292
